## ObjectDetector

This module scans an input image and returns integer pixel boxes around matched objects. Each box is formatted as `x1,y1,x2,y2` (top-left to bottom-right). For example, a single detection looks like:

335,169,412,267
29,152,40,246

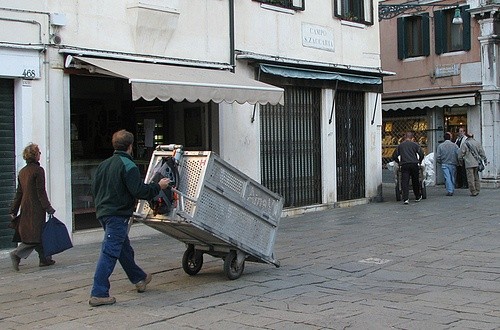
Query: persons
436,132,463,196
455,127,467,148
458,138,487,196
8,144,73,271
88,130,171,307
392,132,424,203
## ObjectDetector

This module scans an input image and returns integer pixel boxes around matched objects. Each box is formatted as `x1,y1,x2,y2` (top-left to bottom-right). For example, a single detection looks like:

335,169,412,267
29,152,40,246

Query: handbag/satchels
479,162,484,172
41,213,74,257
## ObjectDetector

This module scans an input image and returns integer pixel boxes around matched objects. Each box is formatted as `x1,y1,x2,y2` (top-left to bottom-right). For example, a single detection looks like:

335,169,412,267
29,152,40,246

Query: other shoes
470,192,479,196
446,191,454,196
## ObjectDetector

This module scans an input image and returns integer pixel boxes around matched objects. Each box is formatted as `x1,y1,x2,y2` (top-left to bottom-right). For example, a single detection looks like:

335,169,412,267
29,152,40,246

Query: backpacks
147,157,180,214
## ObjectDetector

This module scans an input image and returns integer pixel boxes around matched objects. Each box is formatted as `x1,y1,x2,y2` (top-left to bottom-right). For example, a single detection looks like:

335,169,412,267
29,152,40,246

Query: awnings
69,54,284,107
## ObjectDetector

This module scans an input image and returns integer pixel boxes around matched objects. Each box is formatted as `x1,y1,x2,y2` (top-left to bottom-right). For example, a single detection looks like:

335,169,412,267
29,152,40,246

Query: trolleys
134,144,286,279
394,159,428,201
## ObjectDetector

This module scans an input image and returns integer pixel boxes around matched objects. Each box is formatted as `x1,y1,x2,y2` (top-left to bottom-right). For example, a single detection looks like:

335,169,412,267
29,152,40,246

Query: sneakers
136,272,152,292
39,260,56,267
90,296,116,307
415,195,422,201
405,199,409,204
10,252,19,271
